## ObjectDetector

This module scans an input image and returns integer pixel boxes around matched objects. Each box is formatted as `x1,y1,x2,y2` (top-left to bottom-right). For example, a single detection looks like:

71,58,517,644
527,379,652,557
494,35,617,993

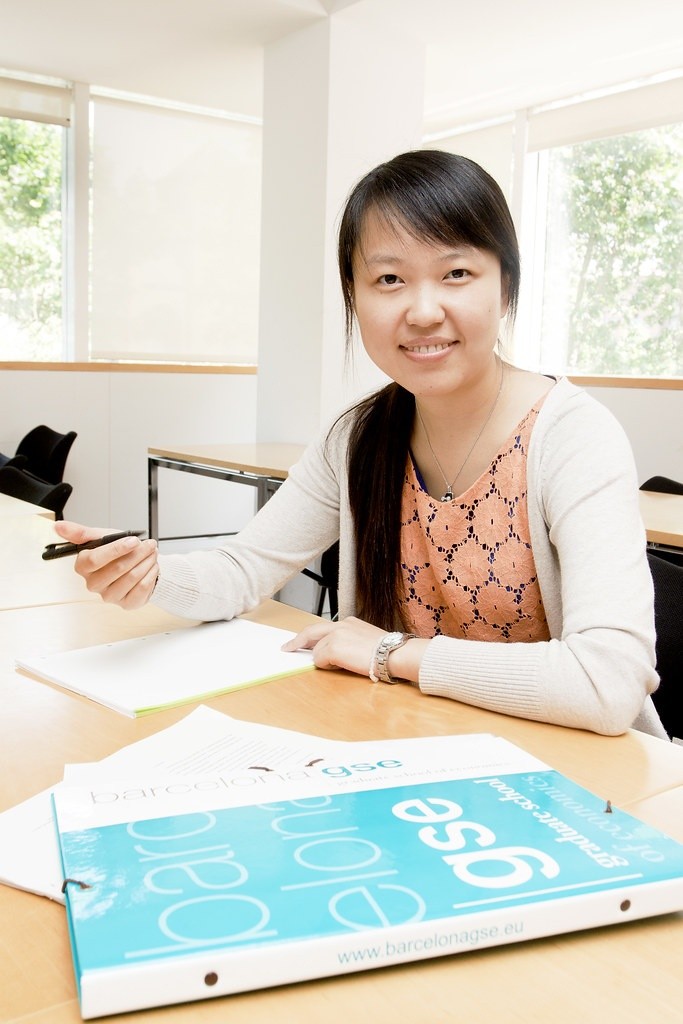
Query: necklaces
416,363,504,503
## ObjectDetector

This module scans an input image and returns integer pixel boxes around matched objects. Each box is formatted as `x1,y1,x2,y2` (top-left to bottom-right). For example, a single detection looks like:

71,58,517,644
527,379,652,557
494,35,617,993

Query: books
16,617,317,719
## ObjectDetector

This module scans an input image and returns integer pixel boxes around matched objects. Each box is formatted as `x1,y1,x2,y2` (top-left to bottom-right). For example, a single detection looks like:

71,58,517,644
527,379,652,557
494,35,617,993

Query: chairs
0,456,73,521
14,424,77,521
646,553,683,742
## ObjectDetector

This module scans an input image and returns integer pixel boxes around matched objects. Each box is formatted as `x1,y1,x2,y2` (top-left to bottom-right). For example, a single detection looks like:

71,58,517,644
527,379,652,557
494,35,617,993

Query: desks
147,440,307,602
0,484,683,1024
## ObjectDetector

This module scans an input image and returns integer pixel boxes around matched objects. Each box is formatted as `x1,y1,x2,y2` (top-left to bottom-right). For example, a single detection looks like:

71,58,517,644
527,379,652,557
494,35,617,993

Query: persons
53,150,670,741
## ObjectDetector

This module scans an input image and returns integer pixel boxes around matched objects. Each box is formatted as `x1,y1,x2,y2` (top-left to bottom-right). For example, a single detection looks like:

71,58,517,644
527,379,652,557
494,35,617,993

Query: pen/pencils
40,527,147,562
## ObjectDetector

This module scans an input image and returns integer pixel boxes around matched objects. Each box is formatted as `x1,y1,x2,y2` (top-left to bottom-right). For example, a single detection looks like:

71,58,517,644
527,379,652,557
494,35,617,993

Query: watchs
377,631,418,685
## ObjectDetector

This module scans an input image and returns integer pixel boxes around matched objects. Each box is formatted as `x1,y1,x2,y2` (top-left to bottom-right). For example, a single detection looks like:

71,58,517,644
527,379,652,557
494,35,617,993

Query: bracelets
369,633,391,683
